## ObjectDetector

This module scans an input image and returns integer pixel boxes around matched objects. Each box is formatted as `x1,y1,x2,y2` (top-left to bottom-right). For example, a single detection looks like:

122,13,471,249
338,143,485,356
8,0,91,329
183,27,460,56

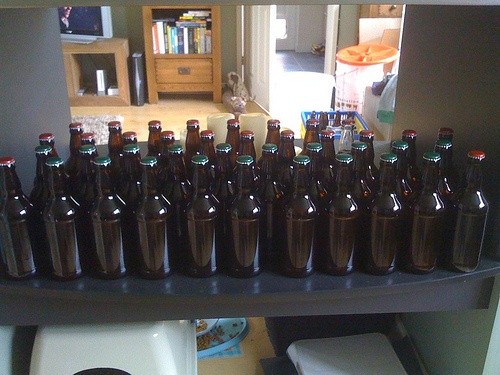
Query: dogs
228,72,249,103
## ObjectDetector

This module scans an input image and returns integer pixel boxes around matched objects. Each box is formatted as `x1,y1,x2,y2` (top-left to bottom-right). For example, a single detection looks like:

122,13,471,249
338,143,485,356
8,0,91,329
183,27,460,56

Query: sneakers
225,73,250,102
222,88,246,114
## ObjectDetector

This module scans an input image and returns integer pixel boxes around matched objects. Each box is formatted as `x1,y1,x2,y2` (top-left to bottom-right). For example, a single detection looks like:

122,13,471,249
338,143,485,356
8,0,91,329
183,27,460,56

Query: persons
59,7,73,30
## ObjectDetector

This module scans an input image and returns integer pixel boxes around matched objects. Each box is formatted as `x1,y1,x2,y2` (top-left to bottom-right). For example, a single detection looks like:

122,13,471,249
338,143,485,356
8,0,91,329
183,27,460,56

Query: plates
196,319,219,337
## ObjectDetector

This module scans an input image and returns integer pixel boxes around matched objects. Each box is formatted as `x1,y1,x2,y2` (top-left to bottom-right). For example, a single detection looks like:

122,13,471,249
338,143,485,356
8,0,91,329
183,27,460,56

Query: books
152,9,212,54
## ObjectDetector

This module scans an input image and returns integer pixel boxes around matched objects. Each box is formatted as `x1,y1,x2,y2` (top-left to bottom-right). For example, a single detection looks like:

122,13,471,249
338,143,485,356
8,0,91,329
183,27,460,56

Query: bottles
37,120,455,279
452,150,491,272
0,156,43,282
305,110,360,132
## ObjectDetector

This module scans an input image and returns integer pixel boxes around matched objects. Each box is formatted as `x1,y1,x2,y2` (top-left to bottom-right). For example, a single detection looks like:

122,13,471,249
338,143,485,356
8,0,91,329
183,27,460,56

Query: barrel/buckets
334,44,400,122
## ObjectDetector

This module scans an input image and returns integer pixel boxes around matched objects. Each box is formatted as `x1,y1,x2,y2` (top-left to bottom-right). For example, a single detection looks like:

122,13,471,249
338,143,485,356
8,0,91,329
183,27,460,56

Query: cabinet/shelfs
62,38,132,107
142,4,222,104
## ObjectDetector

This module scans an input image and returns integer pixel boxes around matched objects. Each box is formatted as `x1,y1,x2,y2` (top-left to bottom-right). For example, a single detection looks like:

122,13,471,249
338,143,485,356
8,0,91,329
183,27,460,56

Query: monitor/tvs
57,6,113,44
